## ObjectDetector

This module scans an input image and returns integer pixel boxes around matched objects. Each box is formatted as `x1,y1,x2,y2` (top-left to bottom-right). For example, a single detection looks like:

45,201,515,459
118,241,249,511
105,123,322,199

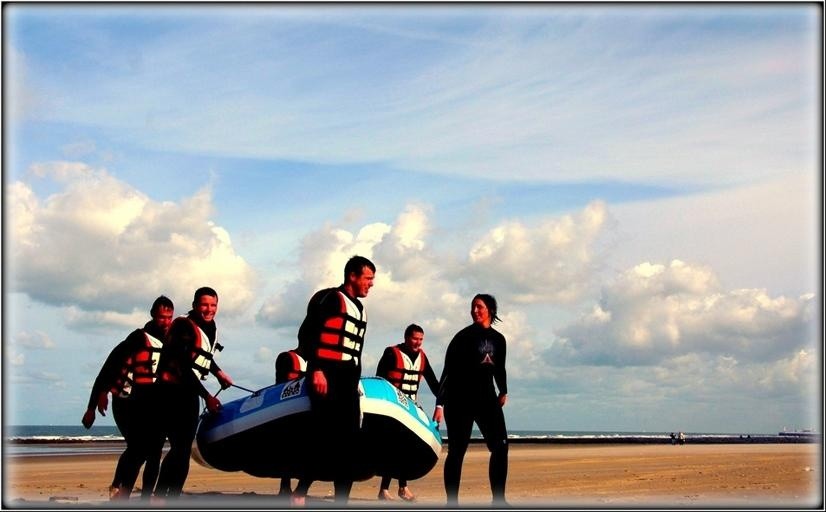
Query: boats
779,426,821,439
189,374,441,482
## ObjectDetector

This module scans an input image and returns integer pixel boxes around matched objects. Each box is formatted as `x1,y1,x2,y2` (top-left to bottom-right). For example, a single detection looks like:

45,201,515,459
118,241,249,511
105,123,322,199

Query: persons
678,431,685,445
670,432,676,445
432,293,511,508
375,324,439,501
151,286,233,497
275,346,308,492
81,340,130,501
289,255,377,507
97,294,175,501
